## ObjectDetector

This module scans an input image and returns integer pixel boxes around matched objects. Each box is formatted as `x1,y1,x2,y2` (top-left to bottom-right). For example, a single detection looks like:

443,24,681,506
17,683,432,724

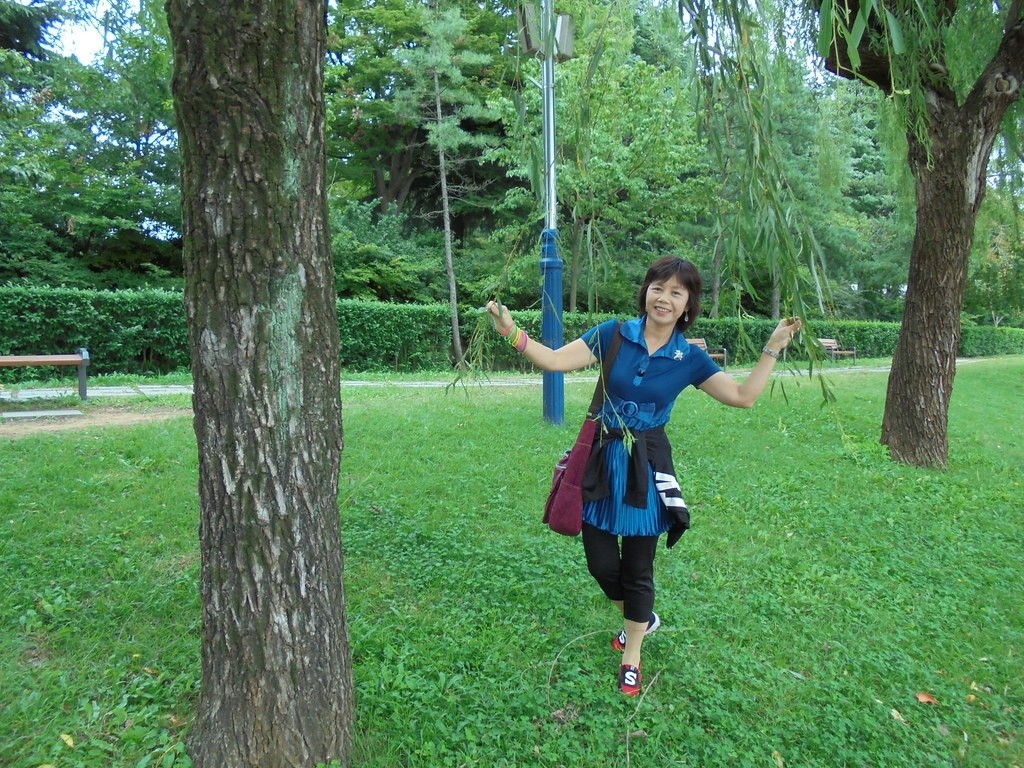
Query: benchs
685,337,726,373
0,347,90,400
812,338,856,365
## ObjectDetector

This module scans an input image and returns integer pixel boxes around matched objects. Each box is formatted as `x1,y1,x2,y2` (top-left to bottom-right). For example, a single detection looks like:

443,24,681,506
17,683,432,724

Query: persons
486,255,801,697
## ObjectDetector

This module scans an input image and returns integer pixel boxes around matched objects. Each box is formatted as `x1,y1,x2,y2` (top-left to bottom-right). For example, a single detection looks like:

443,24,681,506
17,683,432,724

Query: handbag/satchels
542,420,596,537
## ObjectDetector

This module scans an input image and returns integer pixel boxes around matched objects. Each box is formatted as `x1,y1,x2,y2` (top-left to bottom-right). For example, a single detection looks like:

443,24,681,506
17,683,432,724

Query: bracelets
502,321,528,353
762,346,779,359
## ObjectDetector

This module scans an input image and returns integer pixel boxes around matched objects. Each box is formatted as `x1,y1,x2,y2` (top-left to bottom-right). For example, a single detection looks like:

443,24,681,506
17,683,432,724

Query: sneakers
618,659,642,698
610,612,659,650
